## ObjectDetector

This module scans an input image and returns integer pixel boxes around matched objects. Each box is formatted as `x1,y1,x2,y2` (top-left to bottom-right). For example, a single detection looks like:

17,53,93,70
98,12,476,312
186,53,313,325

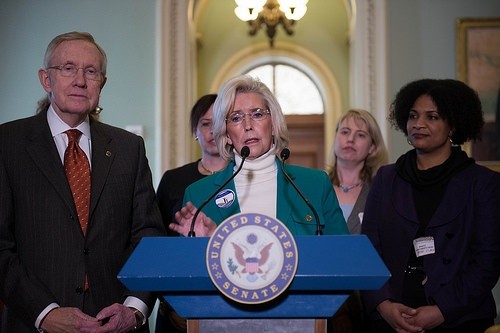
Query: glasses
45,64,105,81
226,108,271,124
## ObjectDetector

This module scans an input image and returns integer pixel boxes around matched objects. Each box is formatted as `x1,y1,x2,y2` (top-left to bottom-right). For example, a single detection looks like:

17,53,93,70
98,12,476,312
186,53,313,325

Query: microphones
281,147,322,236
188,146,250,237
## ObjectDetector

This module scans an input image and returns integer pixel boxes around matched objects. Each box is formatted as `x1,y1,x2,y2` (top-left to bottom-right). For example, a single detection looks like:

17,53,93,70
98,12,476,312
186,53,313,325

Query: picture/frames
455,17,500,173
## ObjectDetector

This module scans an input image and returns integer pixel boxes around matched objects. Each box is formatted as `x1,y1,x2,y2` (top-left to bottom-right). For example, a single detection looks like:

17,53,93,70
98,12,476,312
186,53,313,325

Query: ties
62,129,91,238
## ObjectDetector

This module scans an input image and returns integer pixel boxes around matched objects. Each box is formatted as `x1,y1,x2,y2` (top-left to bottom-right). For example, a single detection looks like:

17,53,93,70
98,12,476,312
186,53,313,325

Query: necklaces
338,181,360,192
201,159,227,175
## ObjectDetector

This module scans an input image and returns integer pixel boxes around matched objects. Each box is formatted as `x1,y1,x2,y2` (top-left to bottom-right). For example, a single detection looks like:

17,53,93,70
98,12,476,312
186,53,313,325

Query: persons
0,31,500,333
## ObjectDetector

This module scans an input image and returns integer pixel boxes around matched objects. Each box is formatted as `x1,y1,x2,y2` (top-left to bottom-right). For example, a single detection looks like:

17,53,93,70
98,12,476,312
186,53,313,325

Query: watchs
128,307,144,329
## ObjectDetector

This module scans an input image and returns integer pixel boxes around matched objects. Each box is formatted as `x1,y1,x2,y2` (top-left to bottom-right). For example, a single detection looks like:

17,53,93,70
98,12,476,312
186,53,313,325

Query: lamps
234,0,308,48
